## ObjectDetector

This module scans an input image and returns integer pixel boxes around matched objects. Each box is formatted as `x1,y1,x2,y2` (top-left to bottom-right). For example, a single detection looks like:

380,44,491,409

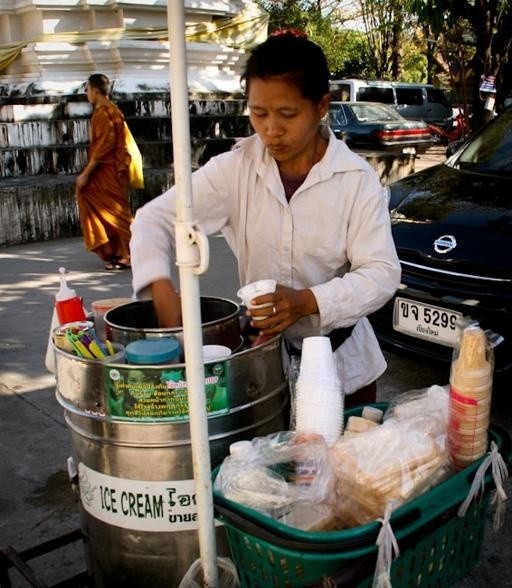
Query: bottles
55,276,85,326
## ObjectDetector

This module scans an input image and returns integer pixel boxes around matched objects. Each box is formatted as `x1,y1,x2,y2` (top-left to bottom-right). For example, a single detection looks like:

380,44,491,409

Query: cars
323,101,432,158
366,101,512,377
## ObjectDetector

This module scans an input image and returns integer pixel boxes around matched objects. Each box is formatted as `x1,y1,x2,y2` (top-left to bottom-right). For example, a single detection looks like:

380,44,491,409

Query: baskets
210,398,503,588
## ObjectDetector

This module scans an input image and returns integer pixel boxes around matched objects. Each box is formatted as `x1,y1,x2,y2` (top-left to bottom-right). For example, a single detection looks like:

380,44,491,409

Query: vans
328,79,454,125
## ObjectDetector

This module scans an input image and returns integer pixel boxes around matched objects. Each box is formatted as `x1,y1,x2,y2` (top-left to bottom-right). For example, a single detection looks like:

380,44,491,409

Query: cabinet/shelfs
0,92,255,251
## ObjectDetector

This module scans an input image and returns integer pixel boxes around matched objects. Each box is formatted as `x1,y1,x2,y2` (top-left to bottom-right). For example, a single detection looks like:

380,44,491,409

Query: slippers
105,262,131,270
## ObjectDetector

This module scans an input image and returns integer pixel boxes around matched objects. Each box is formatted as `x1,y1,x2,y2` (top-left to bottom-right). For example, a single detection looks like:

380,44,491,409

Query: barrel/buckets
48,327,293,586
104,294,245,353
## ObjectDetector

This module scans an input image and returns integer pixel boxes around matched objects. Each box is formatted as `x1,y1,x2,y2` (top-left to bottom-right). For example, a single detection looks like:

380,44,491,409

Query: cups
236,279,277,322
295,336,344,493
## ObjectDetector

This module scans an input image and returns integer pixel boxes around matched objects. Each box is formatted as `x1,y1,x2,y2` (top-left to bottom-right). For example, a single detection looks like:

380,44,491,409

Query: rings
271,305,277,314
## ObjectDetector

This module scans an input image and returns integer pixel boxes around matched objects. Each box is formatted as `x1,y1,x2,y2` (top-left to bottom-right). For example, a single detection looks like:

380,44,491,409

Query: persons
75,73,135,268
128,32,403,427
